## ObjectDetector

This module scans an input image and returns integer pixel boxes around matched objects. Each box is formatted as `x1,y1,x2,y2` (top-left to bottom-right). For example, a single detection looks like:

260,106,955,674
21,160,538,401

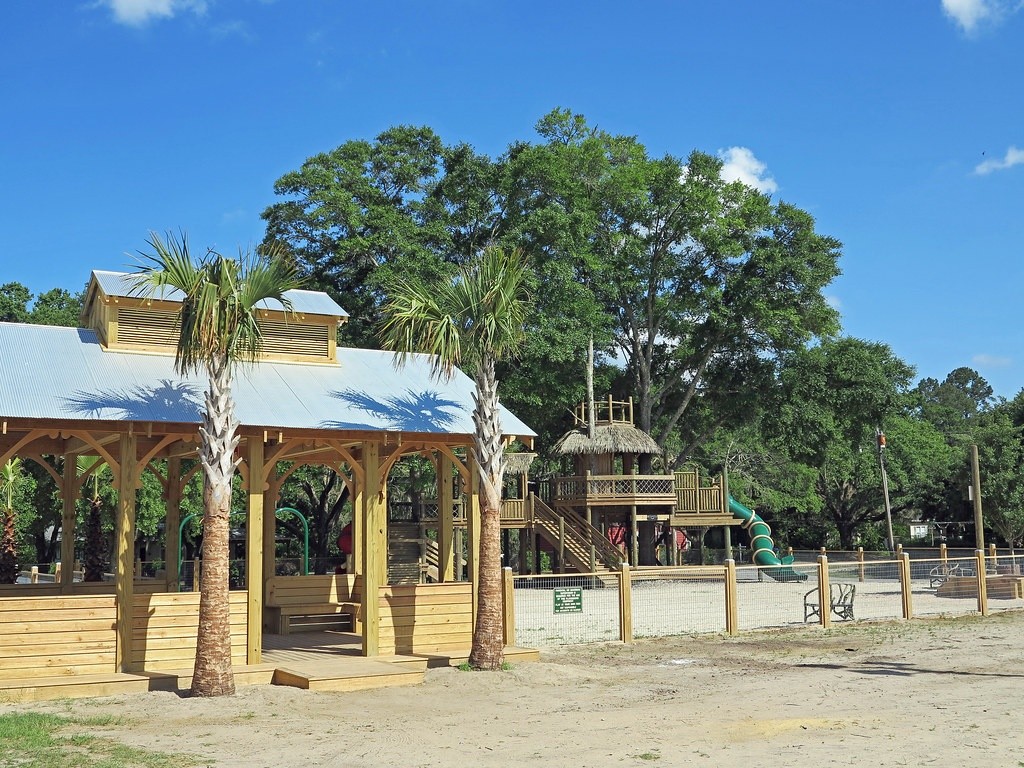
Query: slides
728,492,809,582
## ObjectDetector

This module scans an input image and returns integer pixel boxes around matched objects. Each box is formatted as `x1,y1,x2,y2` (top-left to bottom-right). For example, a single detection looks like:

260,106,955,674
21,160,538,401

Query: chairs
803,578,856,623
929,562,959,589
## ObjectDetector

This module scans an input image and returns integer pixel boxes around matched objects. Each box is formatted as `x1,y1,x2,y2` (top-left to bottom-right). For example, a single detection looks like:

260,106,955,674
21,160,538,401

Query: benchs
1002,575,1024,599
265,574,362,636
